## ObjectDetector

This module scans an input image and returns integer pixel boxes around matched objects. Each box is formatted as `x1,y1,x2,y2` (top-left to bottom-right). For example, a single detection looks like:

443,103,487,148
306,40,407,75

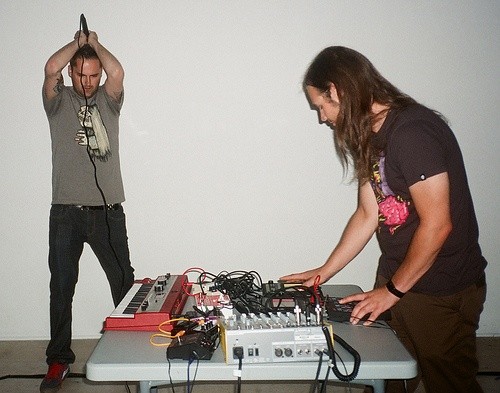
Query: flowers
378,195,410,235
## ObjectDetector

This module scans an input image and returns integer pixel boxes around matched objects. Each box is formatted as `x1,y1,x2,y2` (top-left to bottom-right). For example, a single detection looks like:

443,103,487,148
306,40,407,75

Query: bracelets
383,281,405,299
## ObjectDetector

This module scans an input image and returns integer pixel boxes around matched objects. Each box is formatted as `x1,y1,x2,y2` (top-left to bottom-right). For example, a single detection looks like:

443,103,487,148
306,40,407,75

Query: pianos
100,273,192,332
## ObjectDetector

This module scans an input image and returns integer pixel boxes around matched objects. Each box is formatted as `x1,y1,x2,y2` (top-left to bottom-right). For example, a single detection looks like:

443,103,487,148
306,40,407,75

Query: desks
85,284,418,393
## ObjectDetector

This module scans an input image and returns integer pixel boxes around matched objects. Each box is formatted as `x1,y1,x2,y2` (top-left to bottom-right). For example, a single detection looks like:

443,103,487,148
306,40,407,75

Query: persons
275,46,489,393
38,31,135,393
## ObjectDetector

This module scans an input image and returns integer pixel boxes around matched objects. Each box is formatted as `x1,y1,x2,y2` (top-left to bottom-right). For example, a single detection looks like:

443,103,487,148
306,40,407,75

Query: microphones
80,14,89,44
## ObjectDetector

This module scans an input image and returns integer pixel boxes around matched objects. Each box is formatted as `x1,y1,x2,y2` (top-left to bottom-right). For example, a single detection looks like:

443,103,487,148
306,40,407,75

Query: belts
77,203,119,212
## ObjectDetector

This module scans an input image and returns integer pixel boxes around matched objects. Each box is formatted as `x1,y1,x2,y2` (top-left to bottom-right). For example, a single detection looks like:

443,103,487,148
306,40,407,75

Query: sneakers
36,356,70,393
